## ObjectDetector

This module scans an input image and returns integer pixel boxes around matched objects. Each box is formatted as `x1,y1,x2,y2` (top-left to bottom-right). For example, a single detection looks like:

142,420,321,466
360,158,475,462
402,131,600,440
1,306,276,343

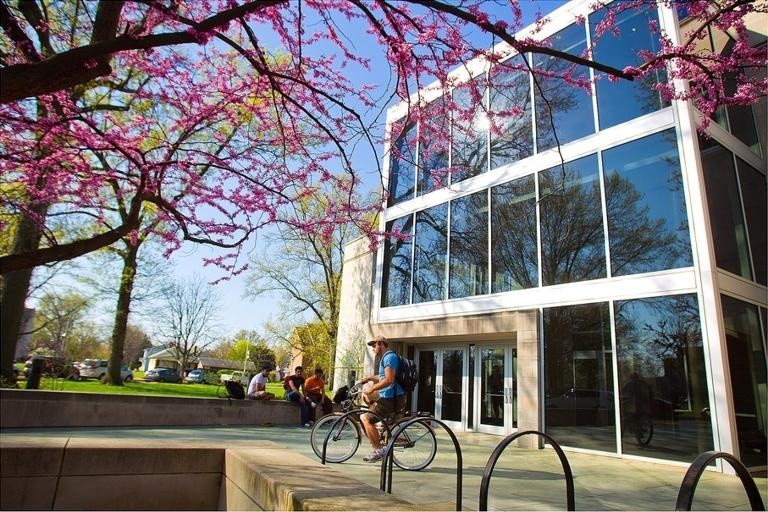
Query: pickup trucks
221,371,250,386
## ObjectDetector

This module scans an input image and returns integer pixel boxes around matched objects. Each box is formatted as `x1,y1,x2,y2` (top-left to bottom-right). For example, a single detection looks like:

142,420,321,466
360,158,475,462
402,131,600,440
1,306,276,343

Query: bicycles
622,399,653,446
311,381,437,471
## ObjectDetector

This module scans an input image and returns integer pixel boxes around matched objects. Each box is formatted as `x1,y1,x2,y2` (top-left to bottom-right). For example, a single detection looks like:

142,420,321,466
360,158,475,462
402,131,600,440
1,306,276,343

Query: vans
79,358,133,382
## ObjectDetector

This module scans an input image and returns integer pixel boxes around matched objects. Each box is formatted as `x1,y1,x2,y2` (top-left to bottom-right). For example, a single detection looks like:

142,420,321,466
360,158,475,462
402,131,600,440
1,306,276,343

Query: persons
489,365,504,422
621,372,653,417
247,366,273,400
361,336,406,462
304,368,333,423
333,385,350,404
286,366,316,427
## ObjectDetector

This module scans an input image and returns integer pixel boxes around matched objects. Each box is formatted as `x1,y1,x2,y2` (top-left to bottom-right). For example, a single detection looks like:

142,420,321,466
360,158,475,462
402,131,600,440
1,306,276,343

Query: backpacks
333,386,353,404
382,352,417,392
217,379,245,399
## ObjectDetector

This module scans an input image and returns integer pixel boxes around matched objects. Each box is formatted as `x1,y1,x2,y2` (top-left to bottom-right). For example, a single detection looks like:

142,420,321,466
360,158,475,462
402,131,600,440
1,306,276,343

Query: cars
144,369,182,384
545,389,615,426
14,355,80,380
187,370,209,384
653,397,674,419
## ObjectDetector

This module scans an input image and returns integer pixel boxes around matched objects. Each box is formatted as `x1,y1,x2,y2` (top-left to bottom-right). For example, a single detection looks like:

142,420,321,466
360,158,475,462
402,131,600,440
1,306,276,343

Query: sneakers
305,420,314,427
363,449,386,463
261,422,273,426
311,402,317,409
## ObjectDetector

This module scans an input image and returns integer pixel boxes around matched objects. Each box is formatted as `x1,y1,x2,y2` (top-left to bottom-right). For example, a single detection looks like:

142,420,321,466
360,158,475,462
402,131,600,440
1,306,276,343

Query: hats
368,336,389,346
